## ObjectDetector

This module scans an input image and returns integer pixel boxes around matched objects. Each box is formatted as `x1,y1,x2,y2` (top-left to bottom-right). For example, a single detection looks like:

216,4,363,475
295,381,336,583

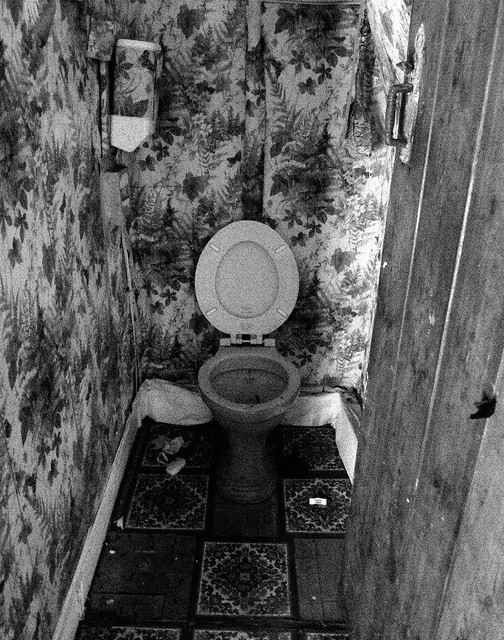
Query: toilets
194,218,301,504
